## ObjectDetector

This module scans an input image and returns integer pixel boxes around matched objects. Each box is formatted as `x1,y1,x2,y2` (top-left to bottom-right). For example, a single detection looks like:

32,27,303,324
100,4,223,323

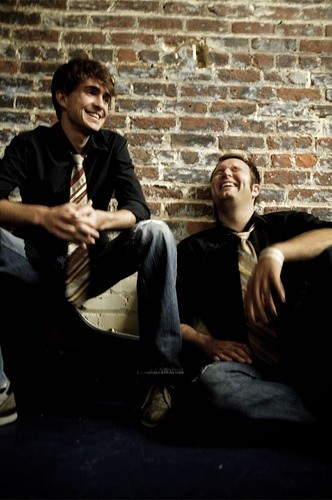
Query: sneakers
0,382,18,426
139,385,172,427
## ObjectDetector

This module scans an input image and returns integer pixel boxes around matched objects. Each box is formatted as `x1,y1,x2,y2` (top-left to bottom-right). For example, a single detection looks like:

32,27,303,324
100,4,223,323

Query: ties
232,224,288,365
65,152,90,309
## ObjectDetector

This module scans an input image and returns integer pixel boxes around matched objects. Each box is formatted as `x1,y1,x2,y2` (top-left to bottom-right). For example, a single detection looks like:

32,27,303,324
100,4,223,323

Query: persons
176,153,332,437
1,59,187,428
